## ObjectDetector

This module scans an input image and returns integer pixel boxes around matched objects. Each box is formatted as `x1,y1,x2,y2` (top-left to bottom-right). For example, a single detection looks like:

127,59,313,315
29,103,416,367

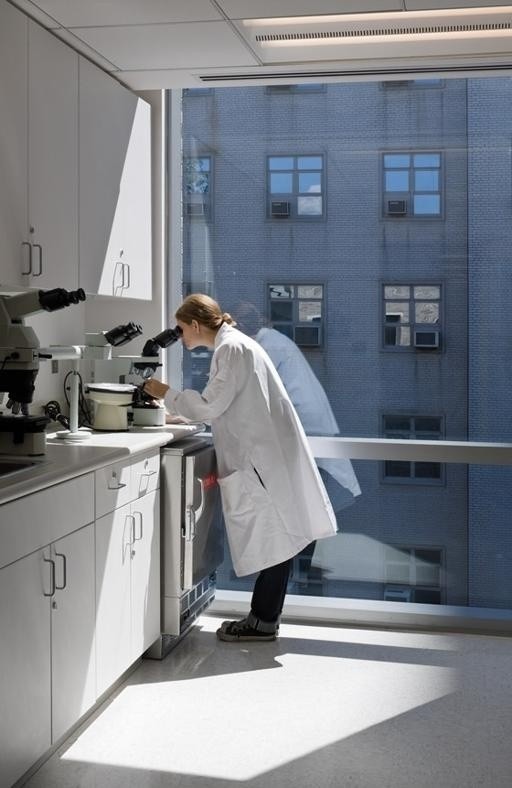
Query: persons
220,295,361,584
142,292,342,640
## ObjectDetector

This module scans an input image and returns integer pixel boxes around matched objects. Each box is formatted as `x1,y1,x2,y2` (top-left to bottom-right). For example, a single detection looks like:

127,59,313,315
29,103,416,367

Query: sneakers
217,619,279,641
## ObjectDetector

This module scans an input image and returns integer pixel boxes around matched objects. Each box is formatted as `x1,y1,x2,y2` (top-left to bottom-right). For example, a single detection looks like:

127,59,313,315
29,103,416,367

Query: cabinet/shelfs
0,471,95,788
78,53,153,302
160,446,225,636
1,1,77,295
96,449,160,697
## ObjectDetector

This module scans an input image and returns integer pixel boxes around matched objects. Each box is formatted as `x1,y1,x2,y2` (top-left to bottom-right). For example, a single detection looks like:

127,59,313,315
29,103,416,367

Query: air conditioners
387,200,408,214
413,330,439,348
293,324,319,347
270,201,289,216
187,202,206,216
383,588,411,602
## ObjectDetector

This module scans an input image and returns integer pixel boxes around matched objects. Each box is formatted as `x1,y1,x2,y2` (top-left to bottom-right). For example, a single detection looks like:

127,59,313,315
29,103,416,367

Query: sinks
0,458,53,479
